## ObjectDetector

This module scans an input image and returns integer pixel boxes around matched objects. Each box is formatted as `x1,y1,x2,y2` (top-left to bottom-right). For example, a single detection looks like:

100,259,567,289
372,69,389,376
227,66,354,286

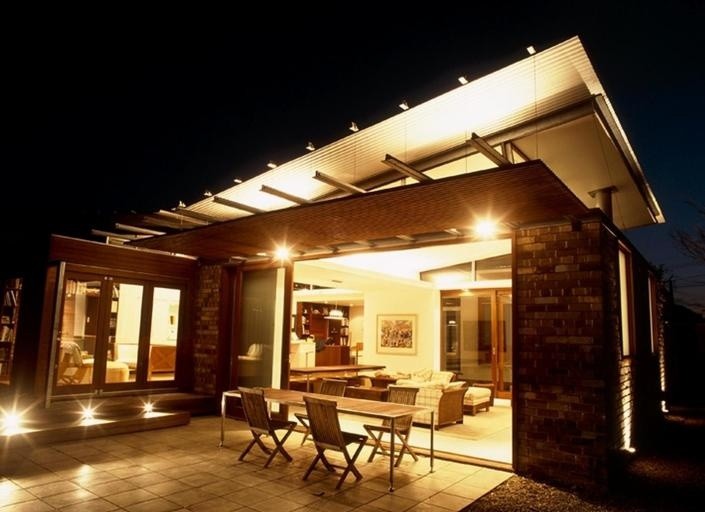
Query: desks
219,386,435,492
290,364,387,393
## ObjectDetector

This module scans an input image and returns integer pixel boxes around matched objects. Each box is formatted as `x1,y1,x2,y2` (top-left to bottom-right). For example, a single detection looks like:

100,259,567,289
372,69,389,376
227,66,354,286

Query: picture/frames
377,314,419,355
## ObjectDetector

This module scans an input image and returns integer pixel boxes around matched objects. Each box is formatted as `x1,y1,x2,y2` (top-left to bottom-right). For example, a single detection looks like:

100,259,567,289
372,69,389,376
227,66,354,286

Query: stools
106,361,130,384
463,387,491,415
473,383,495,406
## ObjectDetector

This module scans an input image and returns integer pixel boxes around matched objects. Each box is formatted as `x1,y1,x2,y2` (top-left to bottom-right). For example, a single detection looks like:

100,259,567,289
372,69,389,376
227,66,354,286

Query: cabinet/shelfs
0,276,24,385
295,301,350,365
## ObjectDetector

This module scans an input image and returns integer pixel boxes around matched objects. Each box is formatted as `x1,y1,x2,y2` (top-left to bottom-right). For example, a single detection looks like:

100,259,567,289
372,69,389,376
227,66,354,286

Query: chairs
238,380,419,490
56,352,97,385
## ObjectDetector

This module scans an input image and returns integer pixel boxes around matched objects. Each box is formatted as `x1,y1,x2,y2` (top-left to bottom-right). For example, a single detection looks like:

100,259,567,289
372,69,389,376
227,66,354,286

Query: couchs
397,368,465,430
